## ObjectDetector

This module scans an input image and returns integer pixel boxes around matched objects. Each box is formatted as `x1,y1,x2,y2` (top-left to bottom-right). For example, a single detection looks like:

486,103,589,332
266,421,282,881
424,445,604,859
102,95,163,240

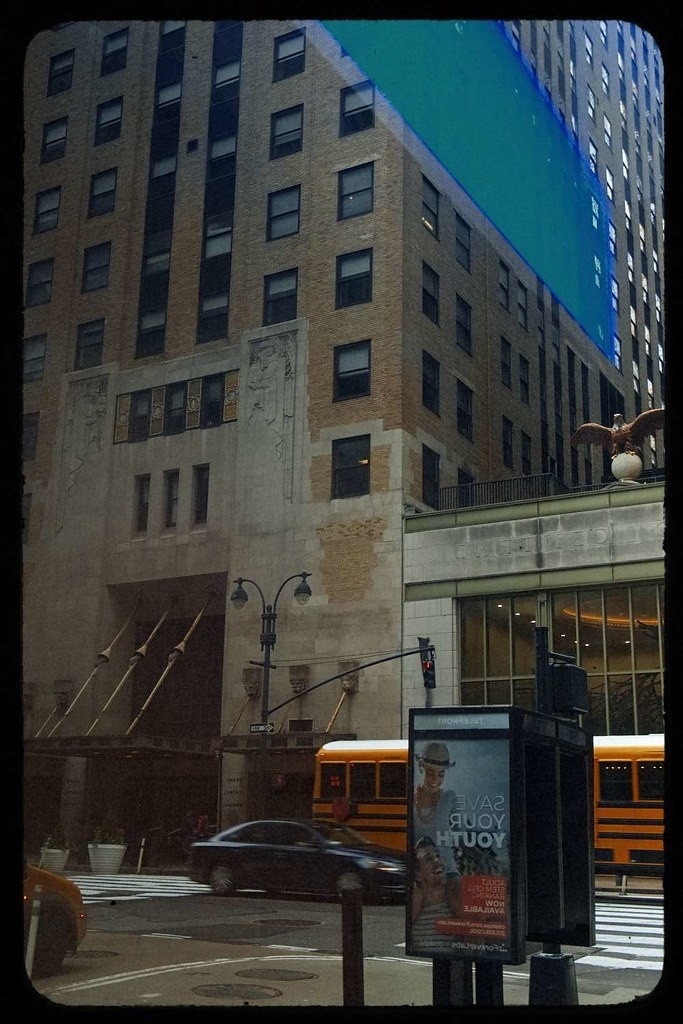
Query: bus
307,732,664,872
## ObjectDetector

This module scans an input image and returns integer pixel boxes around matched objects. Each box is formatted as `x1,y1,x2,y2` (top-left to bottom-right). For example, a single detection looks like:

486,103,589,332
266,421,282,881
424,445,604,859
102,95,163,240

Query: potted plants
87,824,128,876
40,834,75,872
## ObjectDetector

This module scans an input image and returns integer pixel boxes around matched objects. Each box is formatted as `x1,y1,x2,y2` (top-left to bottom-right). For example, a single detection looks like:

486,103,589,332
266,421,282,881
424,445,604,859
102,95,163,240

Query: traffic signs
250,723,275,733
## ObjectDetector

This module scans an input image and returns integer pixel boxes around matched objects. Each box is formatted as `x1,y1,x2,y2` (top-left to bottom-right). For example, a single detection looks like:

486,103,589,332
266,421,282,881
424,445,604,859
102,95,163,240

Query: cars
188,817,410,906
23,854,87,979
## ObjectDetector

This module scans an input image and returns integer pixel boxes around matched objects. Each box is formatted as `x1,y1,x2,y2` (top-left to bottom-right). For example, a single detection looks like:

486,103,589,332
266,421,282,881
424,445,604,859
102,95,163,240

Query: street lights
229,570,313,723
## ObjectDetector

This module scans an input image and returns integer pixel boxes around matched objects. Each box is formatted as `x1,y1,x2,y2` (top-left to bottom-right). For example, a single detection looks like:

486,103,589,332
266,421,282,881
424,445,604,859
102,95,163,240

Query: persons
408,742,496,955
181,808,209,865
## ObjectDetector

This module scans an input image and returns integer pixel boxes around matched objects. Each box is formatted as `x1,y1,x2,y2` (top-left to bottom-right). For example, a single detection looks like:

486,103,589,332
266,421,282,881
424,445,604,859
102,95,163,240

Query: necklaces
416,783,439,822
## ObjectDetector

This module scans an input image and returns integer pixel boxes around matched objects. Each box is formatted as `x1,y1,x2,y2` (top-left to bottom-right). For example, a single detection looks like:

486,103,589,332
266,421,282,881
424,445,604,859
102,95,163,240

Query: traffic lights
420,658,436,689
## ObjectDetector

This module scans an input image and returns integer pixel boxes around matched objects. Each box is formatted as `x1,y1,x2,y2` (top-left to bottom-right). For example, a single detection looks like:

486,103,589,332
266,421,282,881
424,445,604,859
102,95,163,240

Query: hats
417,743,457,770
